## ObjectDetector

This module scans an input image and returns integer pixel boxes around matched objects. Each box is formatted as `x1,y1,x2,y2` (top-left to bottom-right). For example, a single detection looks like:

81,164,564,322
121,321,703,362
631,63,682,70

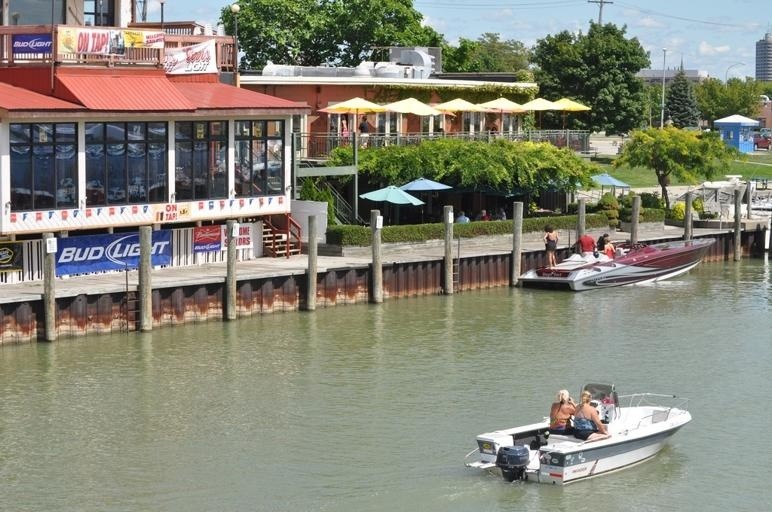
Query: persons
495,207,506,221
544,224,560,267
489,127,497,135
340,115,349,137
573,390,609,441
600,239,616,259
481,208,491,222
549,389,577,435
358,115,370,148
577,233,597,253
455,210,472,223
597,233,610,252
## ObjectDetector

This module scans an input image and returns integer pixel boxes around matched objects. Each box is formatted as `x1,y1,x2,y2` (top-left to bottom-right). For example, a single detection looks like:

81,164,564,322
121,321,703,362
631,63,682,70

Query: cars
754,132,772,151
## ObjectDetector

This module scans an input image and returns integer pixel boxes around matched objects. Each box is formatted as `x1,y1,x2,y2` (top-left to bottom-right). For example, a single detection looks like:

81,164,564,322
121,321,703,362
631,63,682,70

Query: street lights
231,4,240,88
725,62,746,89
661,48,666,129
160,0,165,31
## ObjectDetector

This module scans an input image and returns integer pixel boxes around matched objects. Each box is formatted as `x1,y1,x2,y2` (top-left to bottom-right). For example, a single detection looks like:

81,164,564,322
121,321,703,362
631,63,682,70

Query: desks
752,177,770,190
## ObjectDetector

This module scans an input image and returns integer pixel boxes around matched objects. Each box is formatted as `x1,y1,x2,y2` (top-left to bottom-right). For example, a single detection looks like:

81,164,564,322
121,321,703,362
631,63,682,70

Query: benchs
543,430,587,447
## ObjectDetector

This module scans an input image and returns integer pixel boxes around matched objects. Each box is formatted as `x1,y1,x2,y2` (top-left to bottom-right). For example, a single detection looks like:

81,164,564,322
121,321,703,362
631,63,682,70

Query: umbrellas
429,97,480,131
574,172,631,200
473,97,529,132
313,96,389,133
381,97,441,131
553,97,595,130
357,185,426,224
517,96,566,132
398,177,454,224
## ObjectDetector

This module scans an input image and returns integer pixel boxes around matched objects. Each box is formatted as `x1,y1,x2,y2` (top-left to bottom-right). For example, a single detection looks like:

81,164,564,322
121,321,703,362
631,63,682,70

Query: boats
464,382,692,486
518,237,716,291
